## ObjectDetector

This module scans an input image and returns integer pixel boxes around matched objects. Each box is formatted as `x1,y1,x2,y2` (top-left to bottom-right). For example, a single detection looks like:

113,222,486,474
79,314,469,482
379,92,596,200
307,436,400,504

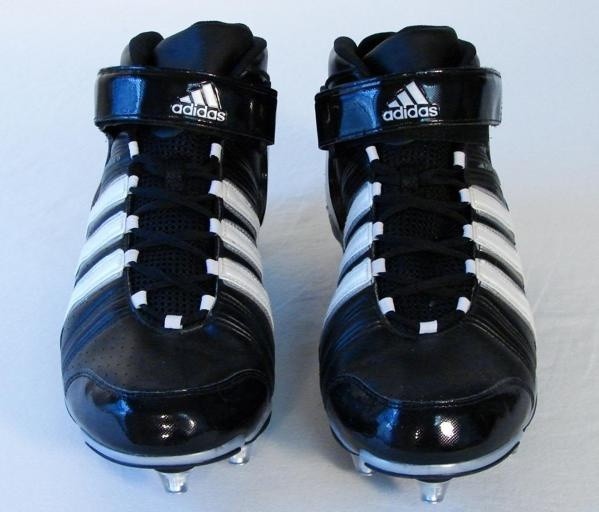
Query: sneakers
314,25,537,504
60,21,277,491
